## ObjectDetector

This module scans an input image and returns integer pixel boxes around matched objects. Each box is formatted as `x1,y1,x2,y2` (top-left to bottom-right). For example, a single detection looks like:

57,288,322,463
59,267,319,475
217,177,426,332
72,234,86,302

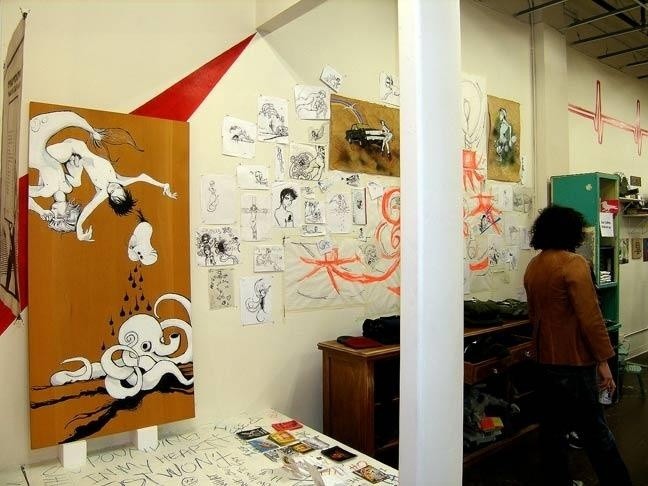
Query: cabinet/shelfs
620,193,646,234
549,172,621,411
318,315,539,480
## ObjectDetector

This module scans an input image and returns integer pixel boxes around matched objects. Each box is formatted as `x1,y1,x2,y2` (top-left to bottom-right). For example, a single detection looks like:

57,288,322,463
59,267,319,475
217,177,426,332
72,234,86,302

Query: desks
22,405,399,485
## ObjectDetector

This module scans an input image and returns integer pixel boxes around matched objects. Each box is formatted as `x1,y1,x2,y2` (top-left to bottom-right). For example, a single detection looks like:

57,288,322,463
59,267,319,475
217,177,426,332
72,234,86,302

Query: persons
274,185,302,230
523,203,634,486
494,107,517,167
